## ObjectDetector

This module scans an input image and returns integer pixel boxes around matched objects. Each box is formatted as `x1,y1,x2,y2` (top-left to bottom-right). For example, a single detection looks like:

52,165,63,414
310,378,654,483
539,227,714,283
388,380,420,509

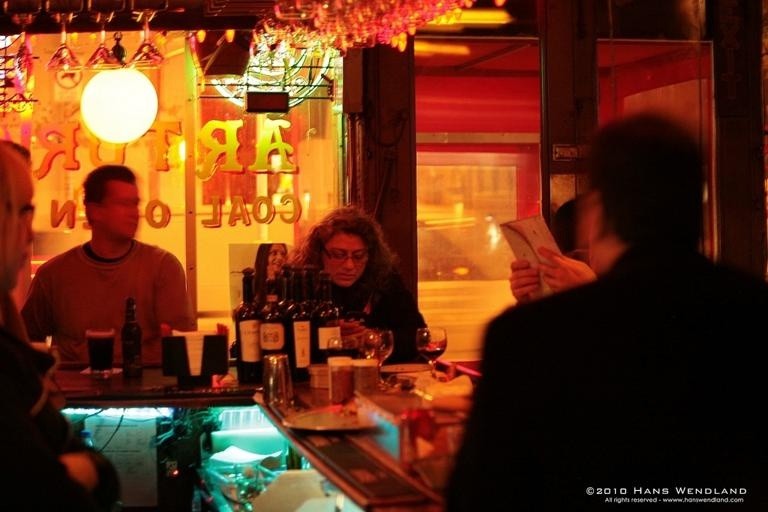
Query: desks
50,363,474,511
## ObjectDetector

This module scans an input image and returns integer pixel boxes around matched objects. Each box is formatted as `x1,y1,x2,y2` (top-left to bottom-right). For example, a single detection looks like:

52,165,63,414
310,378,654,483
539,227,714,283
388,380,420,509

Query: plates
283,405,371,431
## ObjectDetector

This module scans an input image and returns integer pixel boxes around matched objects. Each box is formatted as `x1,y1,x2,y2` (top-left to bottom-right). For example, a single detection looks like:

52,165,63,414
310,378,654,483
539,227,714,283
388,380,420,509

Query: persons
20,164,188,367
1,139,125,511
305,205,432,363
509,188,596,303
247,243,287,278
443,112,768,509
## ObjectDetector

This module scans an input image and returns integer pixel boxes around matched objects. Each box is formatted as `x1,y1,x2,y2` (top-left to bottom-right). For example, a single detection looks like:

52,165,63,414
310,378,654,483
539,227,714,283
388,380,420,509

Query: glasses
324,246,368,261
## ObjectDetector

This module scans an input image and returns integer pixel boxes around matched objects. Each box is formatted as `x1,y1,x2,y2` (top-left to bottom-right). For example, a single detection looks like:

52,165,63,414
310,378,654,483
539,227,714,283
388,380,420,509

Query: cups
327,356,379,402
86,327,116,378
216,411,267,430
327,335,359,358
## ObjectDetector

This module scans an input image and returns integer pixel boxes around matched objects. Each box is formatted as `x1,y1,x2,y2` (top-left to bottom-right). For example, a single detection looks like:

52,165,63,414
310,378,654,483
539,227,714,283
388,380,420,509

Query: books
500,216,562,299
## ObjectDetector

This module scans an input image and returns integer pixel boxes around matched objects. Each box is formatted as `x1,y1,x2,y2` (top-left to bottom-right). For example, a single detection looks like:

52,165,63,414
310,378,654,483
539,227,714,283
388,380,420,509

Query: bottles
399,415,418,465
121,296,141,377
79,430,95,447
263,353,296,406
237,262,342,384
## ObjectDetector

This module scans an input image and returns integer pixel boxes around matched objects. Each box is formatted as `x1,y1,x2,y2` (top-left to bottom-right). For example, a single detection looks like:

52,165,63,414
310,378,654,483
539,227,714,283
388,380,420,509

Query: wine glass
2,0,478,78
365,330,395,390
415,325,448,371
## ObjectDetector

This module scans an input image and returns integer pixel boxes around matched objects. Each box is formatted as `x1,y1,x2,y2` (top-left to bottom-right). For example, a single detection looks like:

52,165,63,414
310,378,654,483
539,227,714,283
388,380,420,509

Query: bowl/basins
207,462,275,502
